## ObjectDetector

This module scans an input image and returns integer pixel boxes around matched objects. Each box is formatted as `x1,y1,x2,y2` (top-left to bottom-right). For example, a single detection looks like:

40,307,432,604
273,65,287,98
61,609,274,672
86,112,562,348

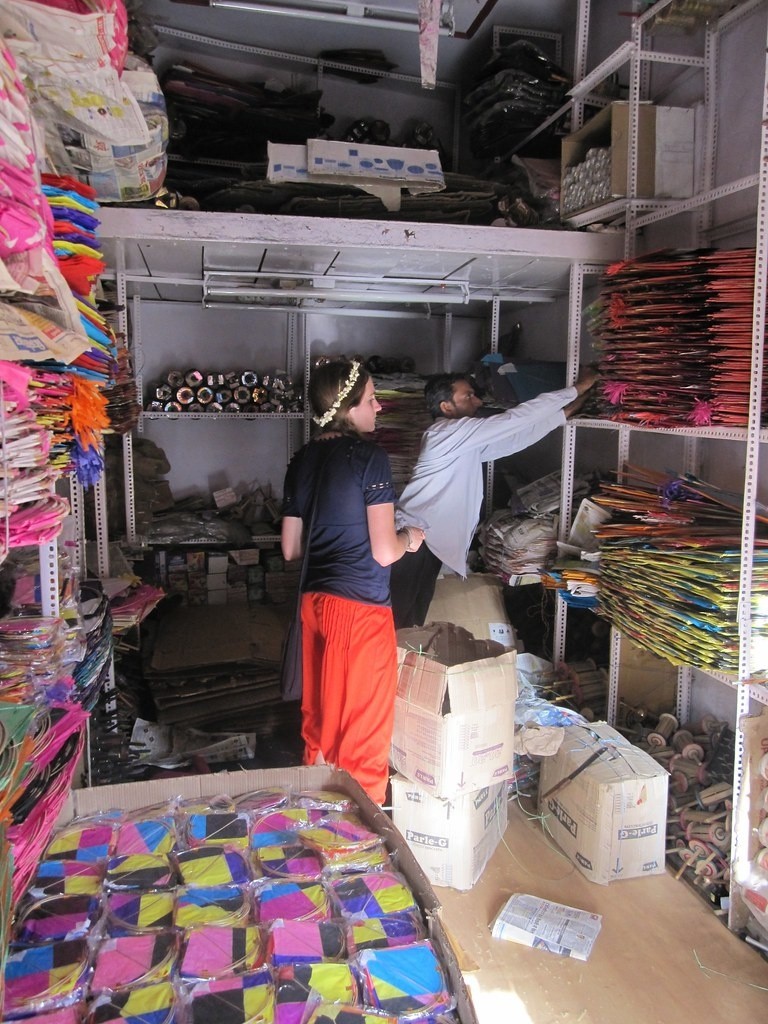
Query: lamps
208,0,455,38
203,296,432,323
200,270,471,306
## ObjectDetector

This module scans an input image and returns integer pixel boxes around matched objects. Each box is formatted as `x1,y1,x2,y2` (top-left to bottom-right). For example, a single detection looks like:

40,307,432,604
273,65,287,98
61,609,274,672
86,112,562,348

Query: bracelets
398,526,415,552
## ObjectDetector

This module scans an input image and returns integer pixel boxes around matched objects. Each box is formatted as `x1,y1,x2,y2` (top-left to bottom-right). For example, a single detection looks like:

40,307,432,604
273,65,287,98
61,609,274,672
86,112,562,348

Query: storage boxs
436,573,519,652
558,101,655,220
535,720,671,886
389,622,518,799
392,772,510,890
0,761,479,1024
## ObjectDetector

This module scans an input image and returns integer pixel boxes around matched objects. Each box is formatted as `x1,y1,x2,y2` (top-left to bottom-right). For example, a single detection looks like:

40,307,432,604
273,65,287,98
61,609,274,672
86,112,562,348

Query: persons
389,365,603,630
279,358,426,809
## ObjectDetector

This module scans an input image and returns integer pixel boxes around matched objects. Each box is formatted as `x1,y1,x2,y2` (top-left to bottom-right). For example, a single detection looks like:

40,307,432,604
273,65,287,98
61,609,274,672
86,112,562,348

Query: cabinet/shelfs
0,0,768,1024
